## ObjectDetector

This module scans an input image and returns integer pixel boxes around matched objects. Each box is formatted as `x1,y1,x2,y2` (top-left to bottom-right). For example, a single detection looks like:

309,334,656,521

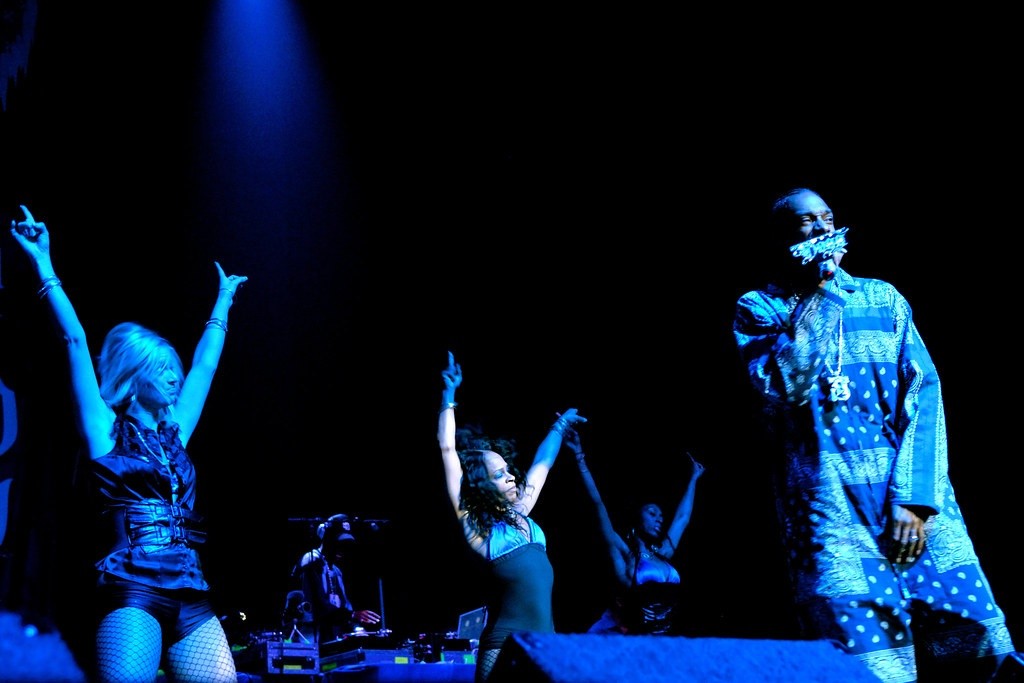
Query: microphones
789,227,849,281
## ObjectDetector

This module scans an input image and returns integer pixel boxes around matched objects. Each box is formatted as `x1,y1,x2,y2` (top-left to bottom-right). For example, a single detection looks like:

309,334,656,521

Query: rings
909,535,919,541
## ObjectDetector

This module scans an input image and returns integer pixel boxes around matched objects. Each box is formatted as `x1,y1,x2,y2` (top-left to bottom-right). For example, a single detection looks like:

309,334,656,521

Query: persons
555,412,705,638
437,351,587,683
282,514,381,644
734,188,1016,683
11,204,248,683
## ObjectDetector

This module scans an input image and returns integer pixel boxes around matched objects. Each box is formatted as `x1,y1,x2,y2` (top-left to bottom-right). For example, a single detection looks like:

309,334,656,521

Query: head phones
316,522,331,543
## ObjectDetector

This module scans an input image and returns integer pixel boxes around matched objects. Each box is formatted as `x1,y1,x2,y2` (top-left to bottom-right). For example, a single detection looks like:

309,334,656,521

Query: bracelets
552,417,573,436
35,276,62,298
440,401,458,412
205,287,235,332
575,451,588,473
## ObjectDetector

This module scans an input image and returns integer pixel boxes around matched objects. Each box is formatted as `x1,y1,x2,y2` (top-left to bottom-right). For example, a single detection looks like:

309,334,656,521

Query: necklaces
641,544,655,559
789,282,851,402
128,419,171,465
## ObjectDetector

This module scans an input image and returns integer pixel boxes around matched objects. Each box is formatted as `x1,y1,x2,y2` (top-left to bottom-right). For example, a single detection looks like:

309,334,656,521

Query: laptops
458,606,488,640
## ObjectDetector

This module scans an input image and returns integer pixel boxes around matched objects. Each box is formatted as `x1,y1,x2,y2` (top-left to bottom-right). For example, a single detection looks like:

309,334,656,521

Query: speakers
485,629,886,683
